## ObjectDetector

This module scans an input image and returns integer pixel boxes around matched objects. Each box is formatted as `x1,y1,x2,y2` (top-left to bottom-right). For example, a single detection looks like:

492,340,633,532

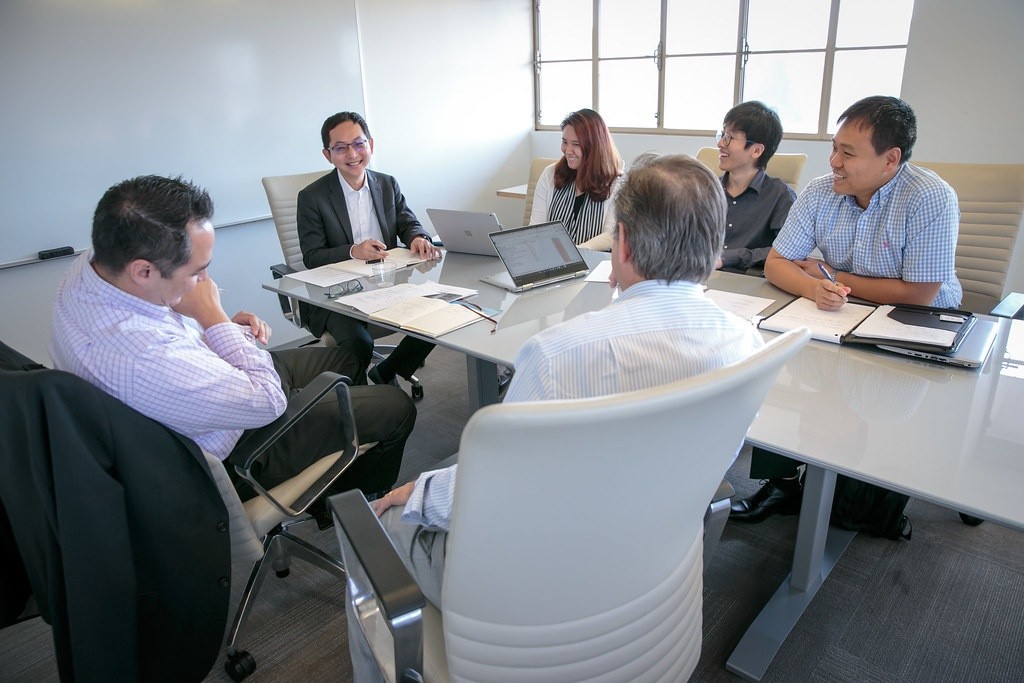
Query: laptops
479,220,589,292
875,319,1000,368
427,210,503,257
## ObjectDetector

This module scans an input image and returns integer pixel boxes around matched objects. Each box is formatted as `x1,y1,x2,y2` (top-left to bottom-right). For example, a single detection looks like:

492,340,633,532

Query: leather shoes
728,478,801,523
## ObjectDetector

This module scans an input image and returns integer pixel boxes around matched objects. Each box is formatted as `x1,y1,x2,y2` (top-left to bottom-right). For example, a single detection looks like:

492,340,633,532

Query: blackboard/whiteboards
0,0,370,273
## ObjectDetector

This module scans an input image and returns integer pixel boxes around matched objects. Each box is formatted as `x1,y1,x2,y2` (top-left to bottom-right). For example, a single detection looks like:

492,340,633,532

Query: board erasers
38,246,74,260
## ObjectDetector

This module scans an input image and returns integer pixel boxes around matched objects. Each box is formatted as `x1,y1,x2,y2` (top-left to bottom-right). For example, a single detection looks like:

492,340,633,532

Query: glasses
715,130,756,146
324,279,363,299
327,138,370,154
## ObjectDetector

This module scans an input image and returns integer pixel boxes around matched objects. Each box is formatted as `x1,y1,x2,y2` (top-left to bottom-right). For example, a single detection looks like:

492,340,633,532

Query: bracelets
831,270,838,282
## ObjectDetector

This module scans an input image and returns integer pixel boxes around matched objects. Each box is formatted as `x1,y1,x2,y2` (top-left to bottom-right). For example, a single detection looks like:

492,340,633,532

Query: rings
389,491,393,496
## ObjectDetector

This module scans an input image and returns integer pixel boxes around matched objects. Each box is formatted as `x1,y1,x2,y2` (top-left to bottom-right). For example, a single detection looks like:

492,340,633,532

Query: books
377,297,491,338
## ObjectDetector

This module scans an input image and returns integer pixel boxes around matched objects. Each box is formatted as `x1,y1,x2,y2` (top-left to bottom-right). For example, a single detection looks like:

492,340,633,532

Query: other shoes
368,363,400,388
497,366,513,395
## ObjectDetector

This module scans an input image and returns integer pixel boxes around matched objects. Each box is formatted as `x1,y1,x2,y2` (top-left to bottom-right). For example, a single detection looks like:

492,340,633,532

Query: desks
261,246,1024,683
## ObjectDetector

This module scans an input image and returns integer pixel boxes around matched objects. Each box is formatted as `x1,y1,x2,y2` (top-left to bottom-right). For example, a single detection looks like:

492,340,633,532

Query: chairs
0,340,378,683
522,157,560,226
327,322,813,682
886,160,1024,540
696,147,808,192
260,169,427,402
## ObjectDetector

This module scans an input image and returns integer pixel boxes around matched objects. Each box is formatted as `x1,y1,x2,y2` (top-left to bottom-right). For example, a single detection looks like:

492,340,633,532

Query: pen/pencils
818,264,847,300
370,238,380,252
218,289,227,292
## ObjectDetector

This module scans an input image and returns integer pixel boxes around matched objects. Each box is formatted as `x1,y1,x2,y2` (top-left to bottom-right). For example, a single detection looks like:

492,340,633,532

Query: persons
372,151,765,611
296,111,443,389
530,109,627,251
714,100,798,271
727,95,963,525
50,174,416,515
